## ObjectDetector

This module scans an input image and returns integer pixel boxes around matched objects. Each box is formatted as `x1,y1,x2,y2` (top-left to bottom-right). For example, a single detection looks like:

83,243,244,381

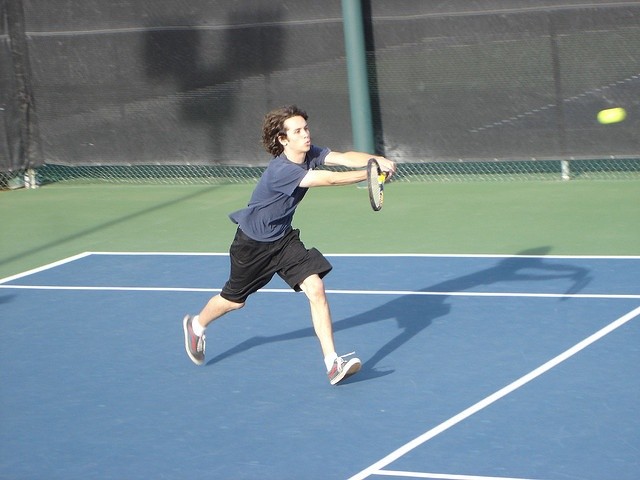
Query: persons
183,102,397,385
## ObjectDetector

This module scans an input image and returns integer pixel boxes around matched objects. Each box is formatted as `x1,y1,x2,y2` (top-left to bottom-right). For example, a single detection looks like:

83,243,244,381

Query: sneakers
182,314,206,365
326,350,362,386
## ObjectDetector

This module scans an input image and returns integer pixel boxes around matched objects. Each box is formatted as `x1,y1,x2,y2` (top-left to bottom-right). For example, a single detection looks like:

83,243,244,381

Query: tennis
596,107,625,124
